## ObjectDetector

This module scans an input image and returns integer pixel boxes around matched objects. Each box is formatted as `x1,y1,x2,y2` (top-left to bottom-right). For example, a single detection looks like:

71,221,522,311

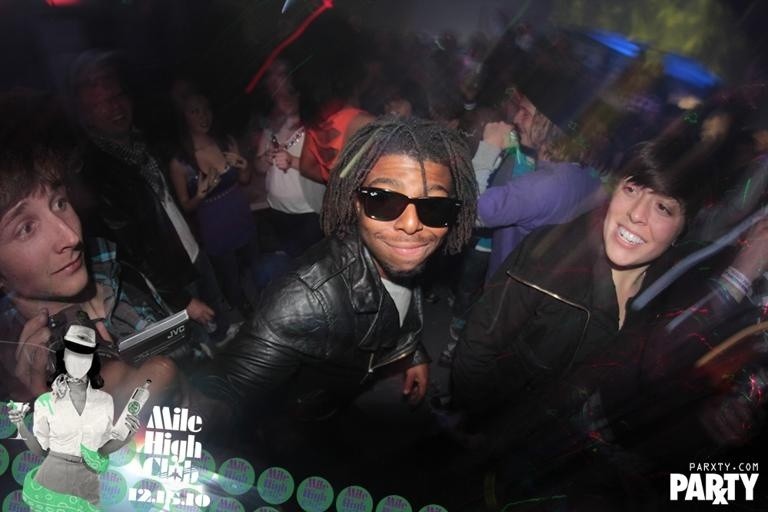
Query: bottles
110,376,152,442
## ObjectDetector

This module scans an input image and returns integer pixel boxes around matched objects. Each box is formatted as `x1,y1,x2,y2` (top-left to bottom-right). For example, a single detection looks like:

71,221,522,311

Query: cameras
44,306,190,376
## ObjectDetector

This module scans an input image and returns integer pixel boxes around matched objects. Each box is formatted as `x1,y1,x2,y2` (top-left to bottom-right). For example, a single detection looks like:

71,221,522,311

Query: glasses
355,186,464,227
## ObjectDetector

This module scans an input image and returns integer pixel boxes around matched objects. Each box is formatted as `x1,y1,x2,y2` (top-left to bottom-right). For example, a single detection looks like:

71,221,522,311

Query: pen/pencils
271,136,288,174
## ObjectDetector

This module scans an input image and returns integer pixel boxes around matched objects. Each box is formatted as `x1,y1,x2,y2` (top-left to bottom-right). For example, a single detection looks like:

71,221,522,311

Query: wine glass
6,402,28,441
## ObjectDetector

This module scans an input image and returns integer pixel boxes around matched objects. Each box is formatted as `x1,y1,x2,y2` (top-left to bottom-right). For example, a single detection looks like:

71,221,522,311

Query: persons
2,0,765,510
